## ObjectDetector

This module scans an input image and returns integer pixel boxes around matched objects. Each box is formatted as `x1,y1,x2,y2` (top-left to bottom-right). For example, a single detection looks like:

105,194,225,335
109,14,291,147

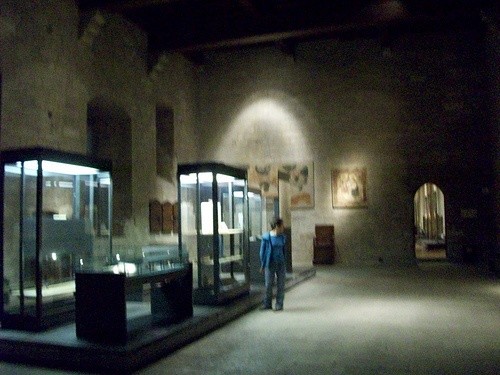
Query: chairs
312,225,334,264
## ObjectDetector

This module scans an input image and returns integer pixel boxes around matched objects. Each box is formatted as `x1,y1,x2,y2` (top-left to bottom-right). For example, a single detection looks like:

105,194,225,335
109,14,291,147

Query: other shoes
274,308,282,311
260,307,272,310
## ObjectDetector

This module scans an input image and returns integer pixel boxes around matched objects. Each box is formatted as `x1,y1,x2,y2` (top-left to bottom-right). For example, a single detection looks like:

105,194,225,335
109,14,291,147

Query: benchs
142,246,168,272
108,250,144,275
167,244,191,266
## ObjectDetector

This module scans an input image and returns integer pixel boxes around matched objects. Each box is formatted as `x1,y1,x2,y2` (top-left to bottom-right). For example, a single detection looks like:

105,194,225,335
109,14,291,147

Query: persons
259,216,288,311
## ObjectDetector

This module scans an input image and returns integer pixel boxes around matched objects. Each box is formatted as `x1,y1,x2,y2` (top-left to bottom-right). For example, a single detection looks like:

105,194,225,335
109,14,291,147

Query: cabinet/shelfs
177,163,249,307
